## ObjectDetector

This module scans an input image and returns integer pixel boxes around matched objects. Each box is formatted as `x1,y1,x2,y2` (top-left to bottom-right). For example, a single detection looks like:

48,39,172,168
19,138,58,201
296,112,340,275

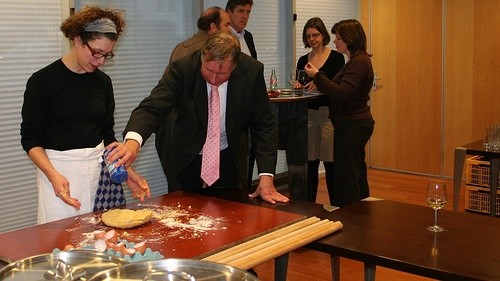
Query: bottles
270,69,278,90
103,148,128,183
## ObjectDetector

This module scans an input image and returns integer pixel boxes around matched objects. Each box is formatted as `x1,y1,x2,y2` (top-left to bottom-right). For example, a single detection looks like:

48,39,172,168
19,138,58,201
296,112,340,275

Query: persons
20,5,151,229
294,16,346,208
303,20,375,206
223,0,258,60
169,7,231,71
106,32,291,205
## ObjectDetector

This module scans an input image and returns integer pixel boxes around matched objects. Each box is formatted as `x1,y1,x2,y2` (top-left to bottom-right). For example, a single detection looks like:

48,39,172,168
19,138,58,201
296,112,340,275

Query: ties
200,85,220,186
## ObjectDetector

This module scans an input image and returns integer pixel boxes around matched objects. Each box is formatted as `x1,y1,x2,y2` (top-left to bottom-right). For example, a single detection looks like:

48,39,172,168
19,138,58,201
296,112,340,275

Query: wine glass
297,70,309,97
288,69,299,97
425,181,447,232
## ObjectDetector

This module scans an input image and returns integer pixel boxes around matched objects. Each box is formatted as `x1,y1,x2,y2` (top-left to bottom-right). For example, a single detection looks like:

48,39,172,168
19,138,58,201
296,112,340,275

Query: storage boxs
464,184,500,216
465,156,500,189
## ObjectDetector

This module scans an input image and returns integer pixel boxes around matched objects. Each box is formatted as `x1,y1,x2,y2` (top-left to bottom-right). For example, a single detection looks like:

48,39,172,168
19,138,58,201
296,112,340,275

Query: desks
1,188,307,281
272,195,500,281
268,86,326,204
451,138,500,217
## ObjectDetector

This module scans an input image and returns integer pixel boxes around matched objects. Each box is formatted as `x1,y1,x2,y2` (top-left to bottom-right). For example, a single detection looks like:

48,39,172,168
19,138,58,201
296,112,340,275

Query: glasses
84,42,115,60
305,32,321,38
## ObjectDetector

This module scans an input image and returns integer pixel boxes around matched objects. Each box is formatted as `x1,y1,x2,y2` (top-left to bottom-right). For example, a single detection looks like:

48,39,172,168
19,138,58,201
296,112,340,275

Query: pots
86,258,262,281
0,250,129,281
